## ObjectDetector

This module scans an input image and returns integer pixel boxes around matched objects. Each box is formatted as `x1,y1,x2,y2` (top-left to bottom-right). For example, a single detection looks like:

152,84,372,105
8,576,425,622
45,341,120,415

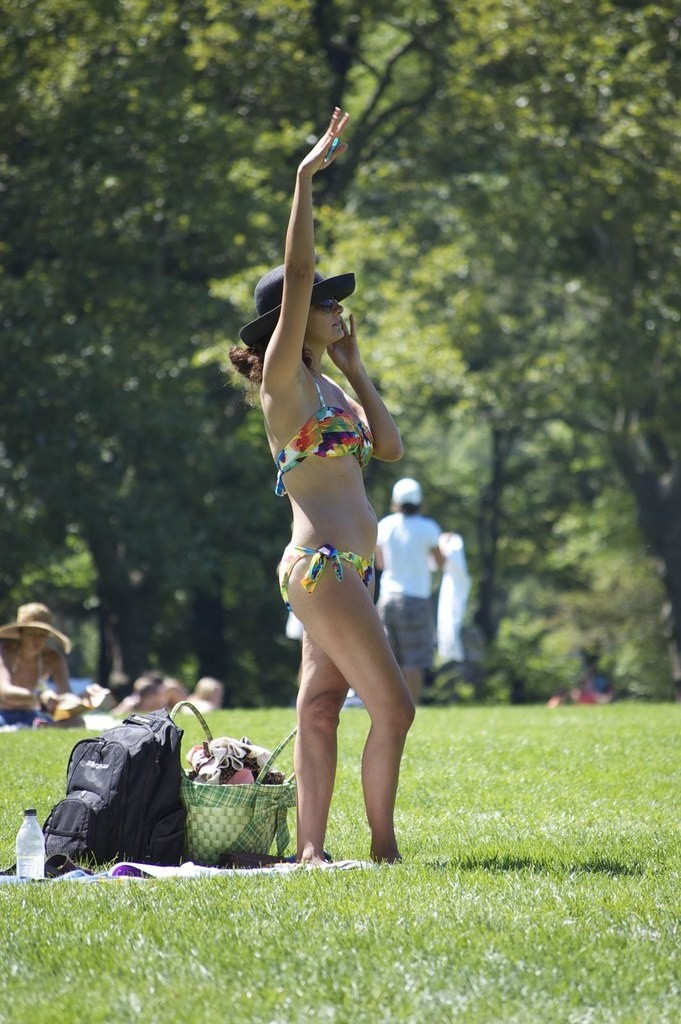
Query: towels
434,532,473,664
186,736,273,785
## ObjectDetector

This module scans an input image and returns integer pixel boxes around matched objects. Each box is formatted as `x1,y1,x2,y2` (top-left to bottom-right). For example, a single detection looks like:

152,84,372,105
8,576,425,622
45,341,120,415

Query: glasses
310,297,338,314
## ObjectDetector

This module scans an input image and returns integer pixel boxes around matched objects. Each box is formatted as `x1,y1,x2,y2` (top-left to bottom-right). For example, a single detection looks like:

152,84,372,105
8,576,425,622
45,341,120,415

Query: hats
238,263,355,347
0,602,70,655
391,477,422,506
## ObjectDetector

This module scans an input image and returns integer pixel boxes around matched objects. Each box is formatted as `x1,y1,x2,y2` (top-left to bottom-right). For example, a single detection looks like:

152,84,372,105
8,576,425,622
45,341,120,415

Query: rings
328,132,335,137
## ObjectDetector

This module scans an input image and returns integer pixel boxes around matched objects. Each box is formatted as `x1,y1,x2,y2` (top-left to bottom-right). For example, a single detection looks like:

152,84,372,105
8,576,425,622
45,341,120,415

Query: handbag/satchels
169,701,298,867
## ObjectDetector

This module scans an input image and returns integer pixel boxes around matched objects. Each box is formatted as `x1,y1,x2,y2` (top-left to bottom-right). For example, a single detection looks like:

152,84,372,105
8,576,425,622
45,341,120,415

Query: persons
82,670,225,718
226,103,418,869
373,476,454,707
1,603,73,732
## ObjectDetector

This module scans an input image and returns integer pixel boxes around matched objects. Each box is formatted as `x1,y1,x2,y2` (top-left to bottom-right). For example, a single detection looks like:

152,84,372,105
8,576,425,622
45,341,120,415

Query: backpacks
46,705,187,867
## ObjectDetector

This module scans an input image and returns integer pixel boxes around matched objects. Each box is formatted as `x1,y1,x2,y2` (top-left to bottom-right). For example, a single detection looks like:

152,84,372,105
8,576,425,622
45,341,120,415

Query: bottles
15,808,45,879
